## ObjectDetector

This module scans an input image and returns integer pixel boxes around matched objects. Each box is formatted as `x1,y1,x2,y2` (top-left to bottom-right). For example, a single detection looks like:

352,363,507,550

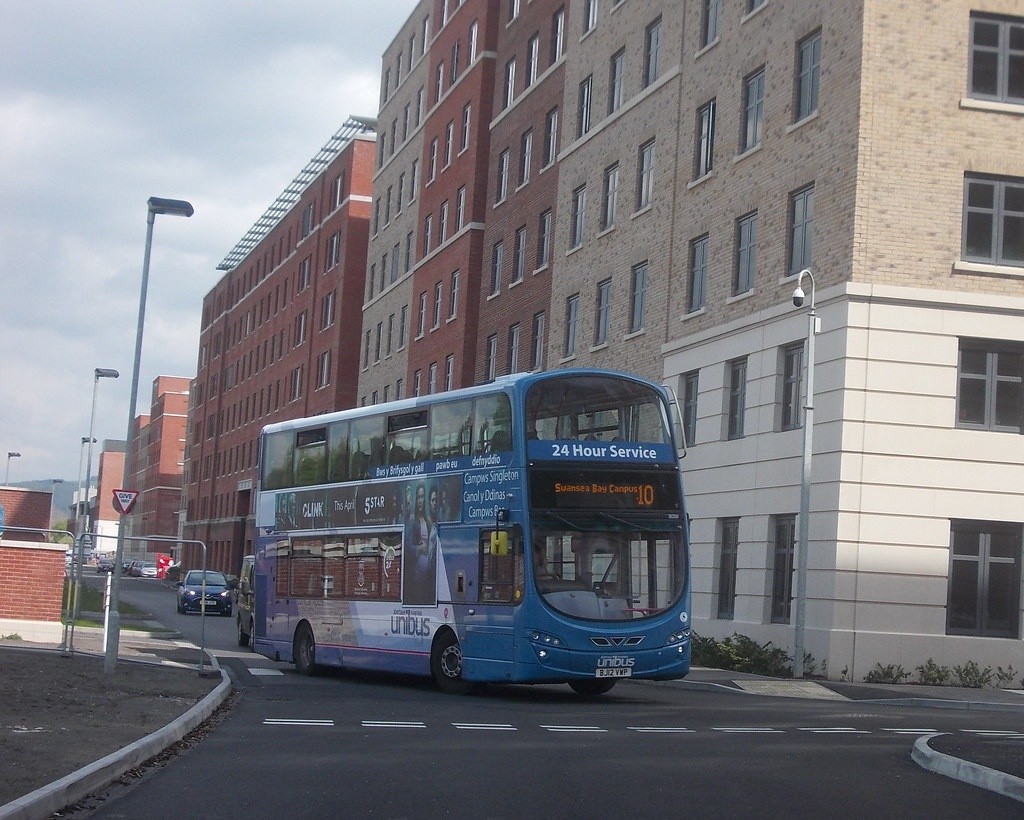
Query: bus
254,370,692,697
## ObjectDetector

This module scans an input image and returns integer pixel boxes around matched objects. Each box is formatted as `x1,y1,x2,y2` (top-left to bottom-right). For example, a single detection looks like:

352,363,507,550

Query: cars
122,560,158,578
227,573,239,588
176,569,233,617
96,559,114,574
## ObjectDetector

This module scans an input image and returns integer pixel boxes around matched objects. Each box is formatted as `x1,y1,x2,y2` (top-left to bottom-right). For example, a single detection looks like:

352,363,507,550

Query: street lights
4,452,21,486
53,480,63,500
102,197,194,674
75,437,97,533
70,368,119,620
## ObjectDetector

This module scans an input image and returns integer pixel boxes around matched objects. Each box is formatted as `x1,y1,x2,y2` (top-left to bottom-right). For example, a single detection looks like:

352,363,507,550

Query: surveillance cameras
792,288,805,307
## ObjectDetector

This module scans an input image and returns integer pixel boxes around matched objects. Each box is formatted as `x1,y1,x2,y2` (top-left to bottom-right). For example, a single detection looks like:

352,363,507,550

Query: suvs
234,555,254,647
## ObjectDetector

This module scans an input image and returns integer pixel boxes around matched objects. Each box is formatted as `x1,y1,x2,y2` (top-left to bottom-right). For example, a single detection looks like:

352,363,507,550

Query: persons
514,537,548,593
387,481,451,579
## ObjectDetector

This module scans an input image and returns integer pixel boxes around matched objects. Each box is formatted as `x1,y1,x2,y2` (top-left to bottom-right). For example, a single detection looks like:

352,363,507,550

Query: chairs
490,430,511,452
352,451,368,478
612,436,625,442
388,447,411,465
282,464,292,487
267,469,286,489
331,448,349,479
296,456,319,483
584,436,597,441
